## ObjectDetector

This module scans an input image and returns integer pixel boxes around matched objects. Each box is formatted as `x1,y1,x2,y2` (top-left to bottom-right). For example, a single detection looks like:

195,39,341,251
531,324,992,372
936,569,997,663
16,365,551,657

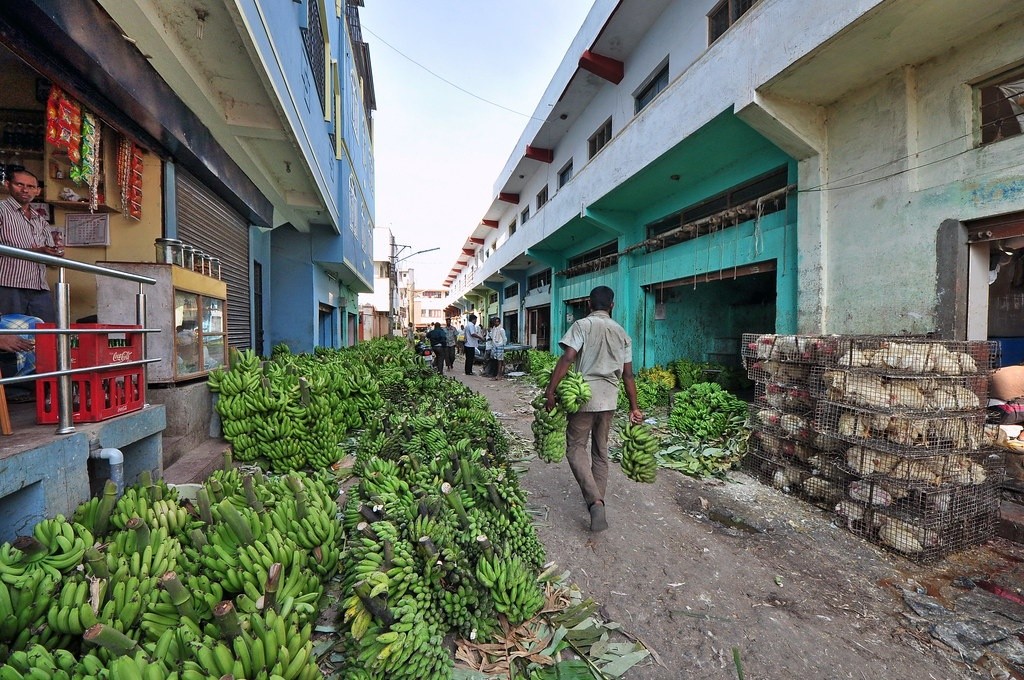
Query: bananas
618,423,659,482
617,357,749,439
331,444,549,680
0,466,343,680
503,348,592,464
206,334,510,476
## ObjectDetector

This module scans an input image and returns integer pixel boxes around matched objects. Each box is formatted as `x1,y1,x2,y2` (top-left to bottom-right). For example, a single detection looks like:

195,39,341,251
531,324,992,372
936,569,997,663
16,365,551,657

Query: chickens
748,333,987,554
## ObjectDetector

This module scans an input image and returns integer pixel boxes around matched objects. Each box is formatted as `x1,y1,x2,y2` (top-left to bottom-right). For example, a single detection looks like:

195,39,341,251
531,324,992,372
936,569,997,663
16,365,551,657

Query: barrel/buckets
988,336,1024,368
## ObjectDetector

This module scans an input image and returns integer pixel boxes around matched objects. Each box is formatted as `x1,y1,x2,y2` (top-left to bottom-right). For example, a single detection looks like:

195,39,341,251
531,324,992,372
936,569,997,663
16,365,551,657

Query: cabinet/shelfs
93,258,230,389
0,107,122,215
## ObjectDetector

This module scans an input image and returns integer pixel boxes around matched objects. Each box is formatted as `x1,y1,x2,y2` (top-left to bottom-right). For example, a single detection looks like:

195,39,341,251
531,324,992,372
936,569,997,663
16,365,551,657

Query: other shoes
468,372,475,375
489,376,502,381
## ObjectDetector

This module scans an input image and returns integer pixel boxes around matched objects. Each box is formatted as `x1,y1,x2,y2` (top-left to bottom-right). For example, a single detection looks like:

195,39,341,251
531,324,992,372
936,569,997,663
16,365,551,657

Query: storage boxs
34,320,146,371
33,366,147,424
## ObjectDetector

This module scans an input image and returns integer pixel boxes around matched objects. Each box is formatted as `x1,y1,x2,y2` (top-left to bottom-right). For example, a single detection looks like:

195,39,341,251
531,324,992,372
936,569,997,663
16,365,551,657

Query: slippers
590,504,608,531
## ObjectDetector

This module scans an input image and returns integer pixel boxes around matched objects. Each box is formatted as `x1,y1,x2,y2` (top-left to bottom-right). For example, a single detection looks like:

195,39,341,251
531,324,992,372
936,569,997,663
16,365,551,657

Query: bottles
194,250,204,275
86,383,91,410
72,385,80,412
14,123,24,148
182,244,194,272
118,381,125,404
46,389,50,412
132,380,138,401
109,339,125,347
9,152,23,172
154,238,182,267
0,151,9,186
75,335,79,348
71,335,74,348
204,254,211,278
26,123,36,150
103,379,110,408
36,124,44,151
3,122,14,148
210,257,221,280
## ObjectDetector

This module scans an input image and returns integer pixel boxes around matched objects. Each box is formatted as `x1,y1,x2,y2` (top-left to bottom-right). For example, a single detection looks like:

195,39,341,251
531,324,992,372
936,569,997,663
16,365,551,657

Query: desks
479,342,531,368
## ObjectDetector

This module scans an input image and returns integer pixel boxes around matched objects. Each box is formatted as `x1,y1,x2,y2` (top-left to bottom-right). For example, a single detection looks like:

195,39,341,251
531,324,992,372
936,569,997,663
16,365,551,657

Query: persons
409,314,507,380
547,286,642,532
0,168,64,404
174,325,198,341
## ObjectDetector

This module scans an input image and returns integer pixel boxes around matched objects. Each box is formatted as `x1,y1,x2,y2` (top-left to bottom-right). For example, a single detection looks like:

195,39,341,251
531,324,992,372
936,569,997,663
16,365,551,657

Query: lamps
324,270,338,282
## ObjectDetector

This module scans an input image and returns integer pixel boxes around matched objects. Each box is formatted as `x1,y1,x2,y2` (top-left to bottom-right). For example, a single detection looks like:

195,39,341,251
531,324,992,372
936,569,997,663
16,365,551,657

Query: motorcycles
413,338,433,367
